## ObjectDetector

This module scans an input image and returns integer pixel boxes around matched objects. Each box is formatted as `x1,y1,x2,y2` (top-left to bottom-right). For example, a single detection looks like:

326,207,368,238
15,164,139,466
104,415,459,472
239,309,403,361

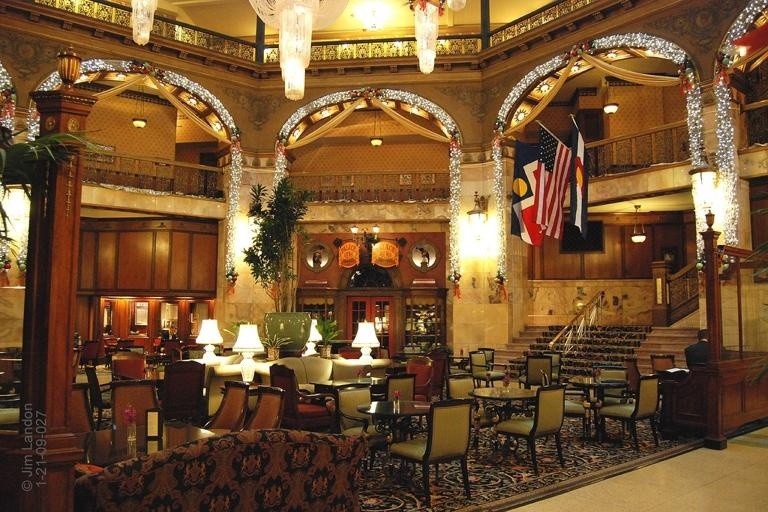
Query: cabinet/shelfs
155,214,189,295
118,216,154,299
295,283,343,338
341,285,400,359
401,284,450,358
77,214,119,299
189,220,217,299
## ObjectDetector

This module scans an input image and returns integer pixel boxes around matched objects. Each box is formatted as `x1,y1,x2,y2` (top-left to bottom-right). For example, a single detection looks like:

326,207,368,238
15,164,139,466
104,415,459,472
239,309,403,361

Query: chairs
0,333,702,512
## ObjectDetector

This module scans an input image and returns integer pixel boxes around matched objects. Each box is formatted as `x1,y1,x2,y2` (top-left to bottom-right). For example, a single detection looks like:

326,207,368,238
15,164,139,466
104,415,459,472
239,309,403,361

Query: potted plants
241,176,319,354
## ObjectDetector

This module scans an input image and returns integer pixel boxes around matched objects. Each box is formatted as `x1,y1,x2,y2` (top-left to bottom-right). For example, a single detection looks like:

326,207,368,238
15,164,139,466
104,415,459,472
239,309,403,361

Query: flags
530,126,572,241
569,117,591,240
511,139,546,247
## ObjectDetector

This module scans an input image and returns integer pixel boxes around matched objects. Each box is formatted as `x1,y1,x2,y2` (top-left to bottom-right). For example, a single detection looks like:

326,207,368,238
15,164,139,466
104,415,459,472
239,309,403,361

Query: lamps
131,84,150,129
466,191,491,240
369,104,382,148
398,0,466,75
195,318,381,367
127,0,161,46
601,77,621,116
631,203,649,244
350,223,380,246
689,144,722,210
249,0,351,103
